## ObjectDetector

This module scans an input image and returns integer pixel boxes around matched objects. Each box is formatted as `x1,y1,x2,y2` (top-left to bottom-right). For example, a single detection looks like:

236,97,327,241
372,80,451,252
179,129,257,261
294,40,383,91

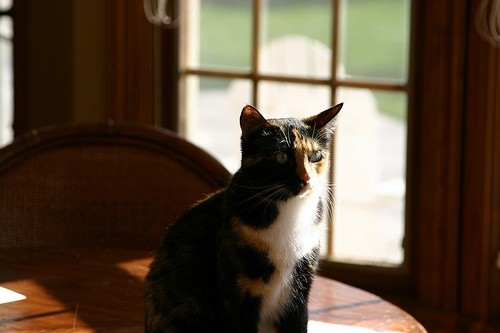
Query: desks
1,249,427,333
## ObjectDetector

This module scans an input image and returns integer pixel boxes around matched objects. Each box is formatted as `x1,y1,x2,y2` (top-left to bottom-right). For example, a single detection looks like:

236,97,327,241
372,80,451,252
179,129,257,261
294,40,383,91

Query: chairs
0,120,233,252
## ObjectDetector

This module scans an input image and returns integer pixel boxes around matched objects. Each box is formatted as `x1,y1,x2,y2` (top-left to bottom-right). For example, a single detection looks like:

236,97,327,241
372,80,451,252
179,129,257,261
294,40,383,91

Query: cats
140,102,344,333
